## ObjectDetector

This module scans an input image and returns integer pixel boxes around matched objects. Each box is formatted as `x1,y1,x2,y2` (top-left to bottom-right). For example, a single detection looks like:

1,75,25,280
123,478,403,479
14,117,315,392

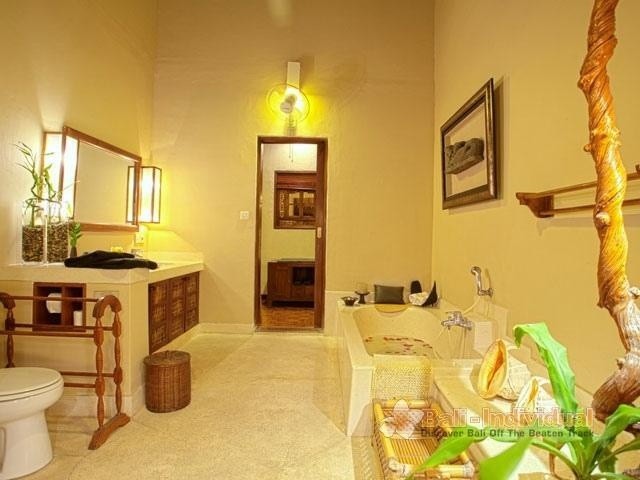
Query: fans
266,61,310,135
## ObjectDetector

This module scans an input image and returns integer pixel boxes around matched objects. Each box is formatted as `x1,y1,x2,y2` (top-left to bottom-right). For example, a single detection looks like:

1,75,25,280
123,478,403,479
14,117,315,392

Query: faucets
131,248,144,260
441,310,475,331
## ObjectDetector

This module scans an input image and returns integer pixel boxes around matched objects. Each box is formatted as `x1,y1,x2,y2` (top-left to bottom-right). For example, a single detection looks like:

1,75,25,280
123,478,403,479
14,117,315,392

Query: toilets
0,365,66,480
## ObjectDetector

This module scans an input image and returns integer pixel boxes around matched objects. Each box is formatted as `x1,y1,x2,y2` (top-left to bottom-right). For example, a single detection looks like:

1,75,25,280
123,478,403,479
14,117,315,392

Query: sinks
156,261,174,267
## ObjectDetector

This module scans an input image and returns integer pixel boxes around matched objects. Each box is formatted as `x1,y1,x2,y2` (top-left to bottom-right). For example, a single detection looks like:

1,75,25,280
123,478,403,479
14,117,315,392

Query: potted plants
46,165,80,262
69,222,83,258
12,140,54,263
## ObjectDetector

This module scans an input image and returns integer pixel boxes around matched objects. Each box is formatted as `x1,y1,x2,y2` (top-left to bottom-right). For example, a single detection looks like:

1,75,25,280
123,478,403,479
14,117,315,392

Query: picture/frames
439,77,497,210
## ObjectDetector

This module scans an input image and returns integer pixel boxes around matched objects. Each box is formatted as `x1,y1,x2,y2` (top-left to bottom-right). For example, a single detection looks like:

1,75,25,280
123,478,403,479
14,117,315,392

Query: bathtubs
340,301,485,369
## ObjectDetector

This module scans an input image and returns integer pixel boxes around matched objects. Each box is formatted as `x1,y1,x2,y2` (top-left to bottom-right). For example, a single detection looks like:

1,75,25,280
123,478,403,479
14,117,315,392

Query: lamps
140,166,162,224
39,131,79,220
126,166,134,223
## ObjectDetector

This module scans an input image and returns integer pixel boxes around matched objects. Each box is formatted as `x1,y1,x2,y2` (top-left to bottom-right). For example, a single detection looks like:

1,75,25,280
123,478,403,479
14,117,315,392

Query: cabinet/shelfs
515,163,640,217
266,261,315,307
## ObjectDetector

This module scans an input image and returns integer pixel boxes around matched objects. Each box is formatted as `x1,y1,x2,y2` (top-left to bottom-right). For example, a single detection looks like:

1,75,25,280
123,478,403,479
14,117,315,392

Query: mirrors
58,127,142,233
